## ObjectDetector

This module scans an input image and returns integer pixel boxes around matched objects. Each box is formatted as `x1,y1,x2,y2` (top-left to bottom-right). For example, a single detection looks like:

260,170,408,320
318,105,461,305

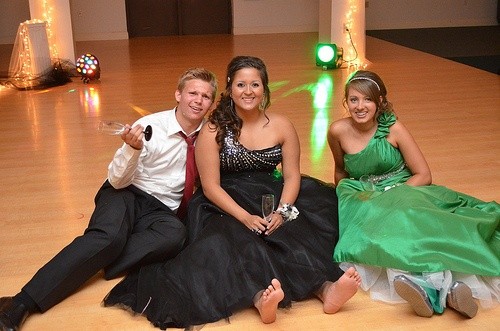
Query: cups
359,175,375,192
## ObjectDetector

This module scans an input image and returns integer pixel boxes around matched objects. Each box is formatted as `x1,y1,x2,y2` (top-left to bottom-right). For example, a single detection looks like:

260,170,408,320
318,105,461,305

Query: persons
0,68,218,331
328,69,500,319
102,55,362,331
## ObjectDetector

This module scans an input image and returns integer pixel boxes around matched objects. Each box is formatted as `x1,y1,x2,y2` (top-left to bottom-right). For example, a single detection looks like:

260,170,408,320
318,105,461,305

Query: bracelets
276,200,299,225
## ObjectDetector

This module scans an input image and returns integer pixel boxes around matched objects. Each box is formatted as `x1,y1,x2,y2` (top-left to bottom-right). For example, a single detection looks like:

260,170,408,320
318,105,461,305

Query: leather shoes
0,296,29,331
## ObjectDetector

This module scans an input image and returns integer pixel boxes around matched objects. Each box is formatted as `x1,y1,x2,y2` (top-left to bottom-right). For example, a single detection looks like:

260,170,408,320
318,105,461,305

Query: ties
176,130,199,221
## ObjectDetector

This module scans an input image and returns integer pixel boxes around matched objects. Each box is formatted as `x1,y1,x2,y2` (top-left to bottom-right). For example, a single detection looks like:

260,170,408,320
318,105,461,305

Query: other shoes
449,281,478,318
393,275,434,318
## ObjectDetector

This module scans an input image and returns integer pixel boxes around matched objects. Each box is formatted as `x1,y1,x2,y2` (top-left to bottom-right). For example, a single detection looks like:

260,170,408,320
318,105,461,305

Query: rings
256,229,259,233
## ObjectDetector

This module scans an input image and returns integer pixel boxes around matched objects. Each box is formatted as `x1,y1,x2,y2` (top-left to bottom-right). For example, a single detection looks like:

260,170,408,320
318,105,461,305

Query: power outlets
344,23,352,33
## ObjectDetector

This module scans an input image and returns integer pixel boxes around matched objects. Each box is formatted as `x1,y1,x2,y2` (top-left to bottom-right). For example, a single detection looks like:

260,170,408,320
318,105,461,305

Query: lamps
77,54,101,85
312,43,344,70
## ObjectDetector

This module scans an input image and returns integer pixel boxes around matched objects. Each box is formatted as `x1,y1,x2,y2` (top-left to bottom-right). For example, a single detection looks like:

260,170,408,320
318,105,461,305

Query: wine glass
98,121,153,141
261,193,274,237
